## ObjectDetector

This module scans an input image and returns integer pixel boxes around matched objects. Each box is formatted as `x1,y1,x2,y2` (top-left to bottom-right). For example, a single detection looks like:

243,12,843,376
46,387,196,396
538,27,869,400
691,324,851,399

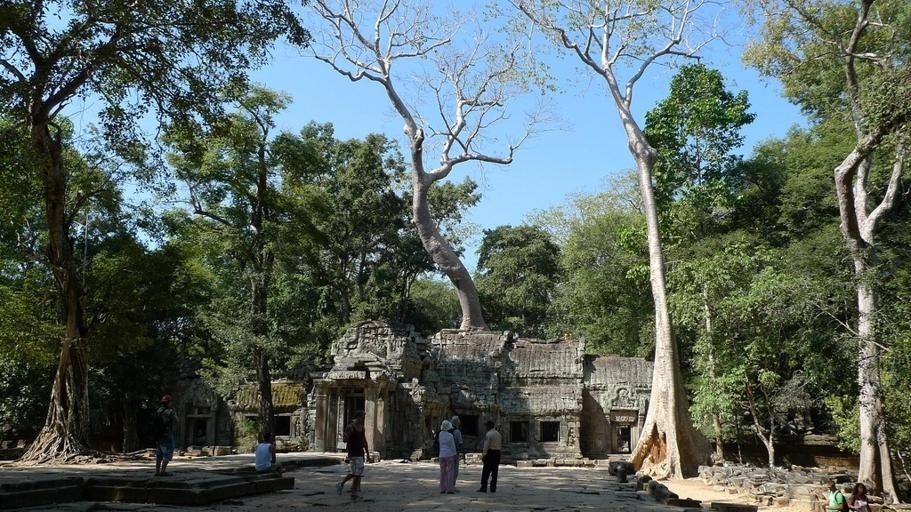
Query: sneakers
336,482,343,495
447,490,456,494
490,490,495,492
477,489,486,493
155,472,173,476
440,491,446,494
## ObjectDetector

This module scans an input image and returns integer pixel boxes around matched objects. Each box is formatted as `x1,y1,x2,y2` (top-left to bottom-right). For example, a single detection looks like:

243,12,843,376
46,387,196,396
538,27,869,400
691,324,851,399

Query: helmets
161,395,172,402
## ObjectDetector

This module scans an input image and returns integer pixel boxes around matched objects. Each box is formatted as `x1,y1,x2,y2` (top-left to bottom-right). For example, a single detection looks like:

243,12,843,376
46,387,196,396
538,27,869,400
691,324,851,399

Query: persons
437,420,459,494
254,429,283,475
477,420,504,492
822,480,845,511
154,395,181,476
449,415,464,486
846,482,870,511
337,410,374,499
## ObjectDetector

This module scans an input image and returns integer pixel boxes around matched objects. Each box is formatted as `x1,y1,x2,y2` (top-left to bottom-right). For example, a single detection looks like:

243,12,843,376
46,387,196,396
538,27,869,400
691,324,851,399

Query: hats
484,421,494,426
441,420,452,431
451,416,460,426
